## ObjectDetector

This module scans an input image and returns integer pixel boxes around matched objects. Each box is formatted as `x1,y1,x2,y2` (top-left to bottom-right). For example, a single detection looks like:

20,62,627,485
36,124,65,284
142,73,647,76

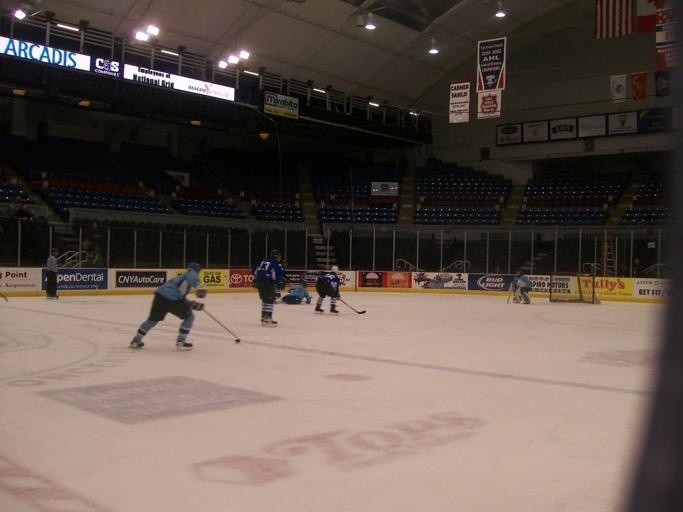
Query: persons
1,202,37,220
315,266,342,313
508,270,533,304
252,248,289,326
275,283,311,304
130,262,207,347
46,248,59,299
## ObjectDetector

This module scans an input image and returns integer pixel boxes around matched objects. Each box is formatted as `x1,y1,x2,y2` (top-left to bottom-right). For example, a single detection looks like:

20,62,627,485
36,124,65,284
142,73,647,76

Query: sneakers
131,340,145,346
261,317,278,324
176,341,193,347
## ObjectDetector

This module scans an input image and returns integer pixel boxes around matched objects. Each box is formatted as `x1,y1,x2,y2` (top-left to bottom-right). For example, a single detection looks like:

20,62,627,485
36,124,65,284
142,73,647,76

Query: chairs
0,133,678,277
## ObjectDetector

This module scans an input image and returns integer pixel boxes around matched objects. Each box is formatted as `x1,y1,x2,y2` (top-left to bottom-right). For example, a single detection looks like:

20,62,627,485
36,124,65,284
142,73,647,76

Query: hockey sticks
203,308,241,343
339,299,366,314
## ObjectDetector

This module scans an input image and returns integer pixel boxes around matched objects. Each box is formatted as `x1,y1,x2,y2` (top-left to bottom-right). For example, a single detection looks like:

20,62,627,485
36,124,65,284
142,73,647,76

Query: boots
331,304,338,312
315,303,324,311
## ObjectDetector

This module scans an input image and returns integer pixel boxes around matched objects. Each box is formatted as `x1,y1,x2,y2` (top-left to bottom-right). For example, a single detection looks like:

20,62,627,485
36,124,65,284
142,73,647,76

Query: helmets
188,262,200,272
332,266,339,272
271,249,280,255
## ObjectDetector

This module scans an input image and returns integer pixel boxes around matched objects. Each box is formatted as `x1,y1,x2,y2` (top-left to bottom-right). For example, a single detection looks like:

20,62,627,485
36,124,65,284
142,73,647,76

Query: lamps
495,1,506,17
355,12,364,26
428,38,439,55
365,13,376,30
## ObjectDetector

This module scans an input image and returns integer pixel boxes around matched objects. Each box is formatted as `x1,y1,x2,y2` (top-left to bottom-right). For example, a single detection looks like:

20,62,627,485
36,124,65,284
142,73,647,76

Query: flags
655,9,683,70
594,0,633,40
636,0,673,33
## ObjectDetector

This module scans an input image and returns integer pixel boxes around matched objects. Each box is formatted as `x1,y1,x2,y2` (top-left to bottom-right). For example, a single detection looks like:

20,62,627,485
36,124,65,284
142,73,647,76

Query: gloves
192,301,204,311
196,284,207,298
